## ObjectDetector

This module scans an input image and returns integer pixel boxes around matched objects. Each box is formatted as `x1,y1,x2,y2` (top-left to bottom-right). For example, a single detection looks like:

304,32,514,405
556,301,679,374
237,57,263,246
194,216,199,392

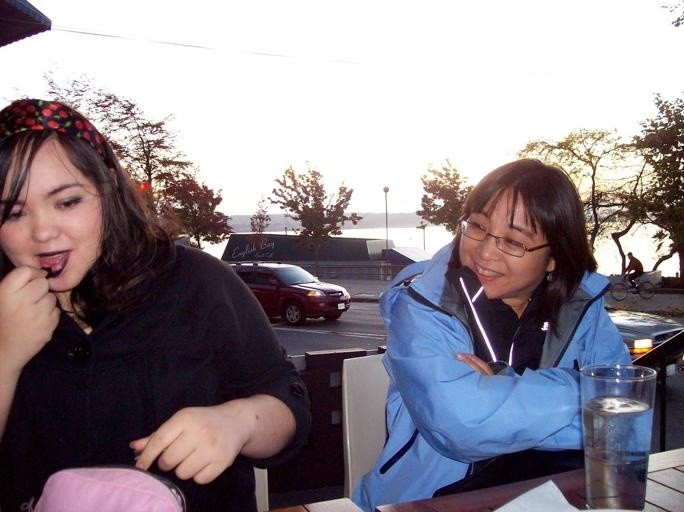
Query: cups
578,362,659,510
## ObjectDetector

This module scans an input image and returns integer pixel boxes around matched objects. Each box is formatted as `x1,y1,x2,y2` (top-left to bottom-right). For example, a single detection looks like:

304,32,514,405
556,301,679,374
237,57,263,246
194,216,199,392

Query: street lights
382,186,389,264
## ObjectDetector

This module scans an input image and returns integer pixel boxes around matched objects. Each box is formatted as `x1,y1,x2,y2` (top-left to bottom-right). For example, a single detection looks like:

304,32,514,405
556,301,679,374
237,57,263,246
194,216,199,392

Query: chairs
340,353,392,502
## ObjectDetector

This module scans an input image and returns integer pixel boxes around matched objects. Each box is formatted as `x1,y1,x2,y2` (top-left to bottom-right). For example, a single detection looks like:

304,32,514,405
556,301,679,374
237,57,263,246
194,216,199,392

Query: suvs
228,260,350,326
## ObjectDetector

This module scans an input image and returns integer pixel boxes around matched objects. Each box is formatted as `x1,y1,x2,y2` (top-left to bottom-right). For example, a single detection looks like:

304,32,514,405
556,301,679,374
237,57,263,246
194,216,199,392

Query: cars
600,303,684,368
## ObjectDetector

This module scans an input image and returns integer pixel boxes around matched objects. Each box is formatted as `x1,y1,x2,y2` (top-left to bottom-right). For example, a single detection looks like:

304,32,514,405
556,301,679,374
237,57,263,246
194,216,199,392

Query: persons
1,98,313,511
621,252,643,294
349,155,635,512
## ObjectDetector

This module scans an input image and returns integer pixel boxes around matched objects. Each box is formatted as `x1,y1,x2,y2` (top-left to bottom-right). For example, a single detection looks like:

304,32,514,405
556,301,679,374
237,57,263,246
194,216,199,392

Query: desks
375,447,684,511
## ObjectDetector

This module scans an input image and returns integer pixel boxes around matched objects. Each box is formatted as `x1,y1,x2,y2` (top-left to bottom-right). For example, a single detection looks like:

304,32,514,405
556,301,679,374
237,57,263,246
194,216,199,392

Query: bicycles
610,270,657,301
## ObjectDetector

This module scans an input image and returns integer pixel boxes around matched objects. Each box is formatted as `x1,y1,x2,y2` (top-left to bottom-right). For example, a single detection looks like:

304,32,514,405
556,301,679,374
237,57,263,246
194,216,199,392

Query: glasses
457,212,550,258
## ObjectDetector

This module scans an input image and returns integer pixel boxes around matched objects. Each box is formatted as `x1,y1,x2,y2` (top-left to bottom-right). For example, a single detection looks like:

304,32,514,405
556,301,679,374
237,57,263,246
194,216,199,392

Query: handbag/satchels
27,465,186,512
